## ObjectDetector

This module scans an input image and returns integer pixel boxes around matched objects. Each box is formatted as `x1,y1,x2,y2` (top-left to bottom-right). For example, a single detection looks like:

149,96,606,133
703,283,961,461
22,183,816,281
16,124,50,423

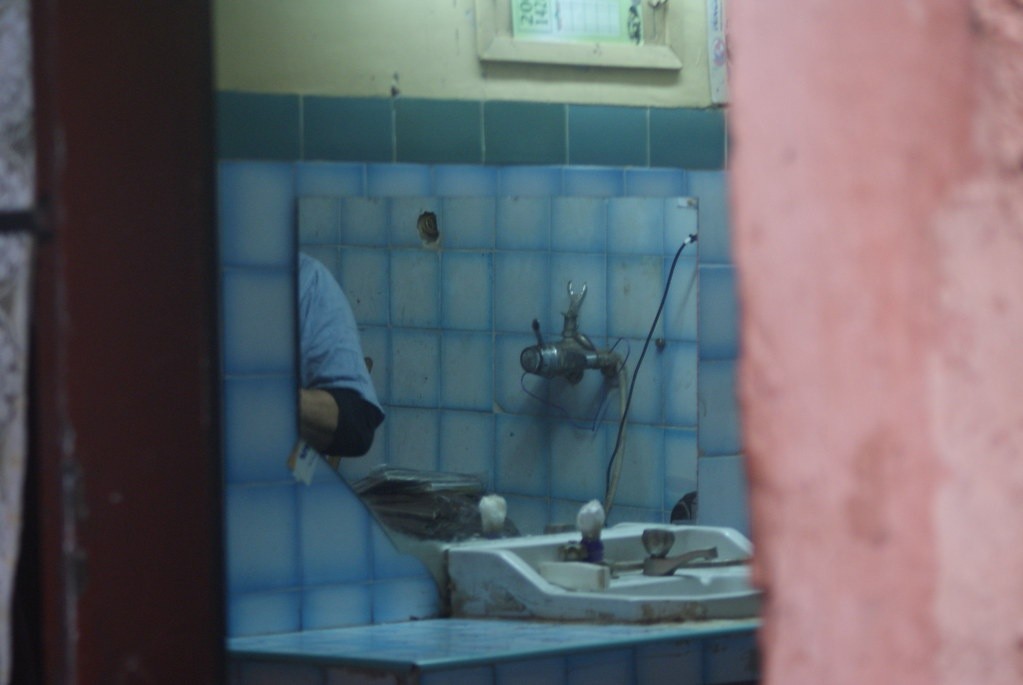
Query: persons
299,250,386,458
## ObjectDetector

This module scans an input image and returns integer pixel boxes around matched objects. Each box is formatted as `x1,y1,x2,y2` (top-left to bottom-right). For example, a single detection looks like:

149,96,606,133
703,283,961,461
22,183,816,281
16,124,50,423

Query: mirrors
295,195,698,598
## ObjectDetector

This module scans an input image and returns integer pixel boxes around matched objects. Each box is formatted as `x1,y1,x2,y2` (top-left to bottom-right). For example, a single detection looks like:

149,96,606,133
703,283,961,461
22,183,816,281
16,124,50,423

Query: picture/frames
473,0,687,71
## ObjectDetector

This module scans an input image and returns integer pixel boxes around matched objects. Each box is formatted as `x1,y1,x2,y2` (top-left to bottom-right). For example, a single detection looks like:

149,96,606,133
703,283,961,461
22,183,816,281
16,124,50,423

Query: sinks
368,506,485,600
448,523,764,623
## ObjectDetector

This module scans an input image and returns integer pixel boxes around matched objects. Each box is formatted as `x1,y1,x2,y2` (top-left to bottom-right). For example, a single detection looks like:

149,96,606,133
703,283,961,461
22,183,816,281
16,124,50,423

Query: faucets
641,529,719,576
